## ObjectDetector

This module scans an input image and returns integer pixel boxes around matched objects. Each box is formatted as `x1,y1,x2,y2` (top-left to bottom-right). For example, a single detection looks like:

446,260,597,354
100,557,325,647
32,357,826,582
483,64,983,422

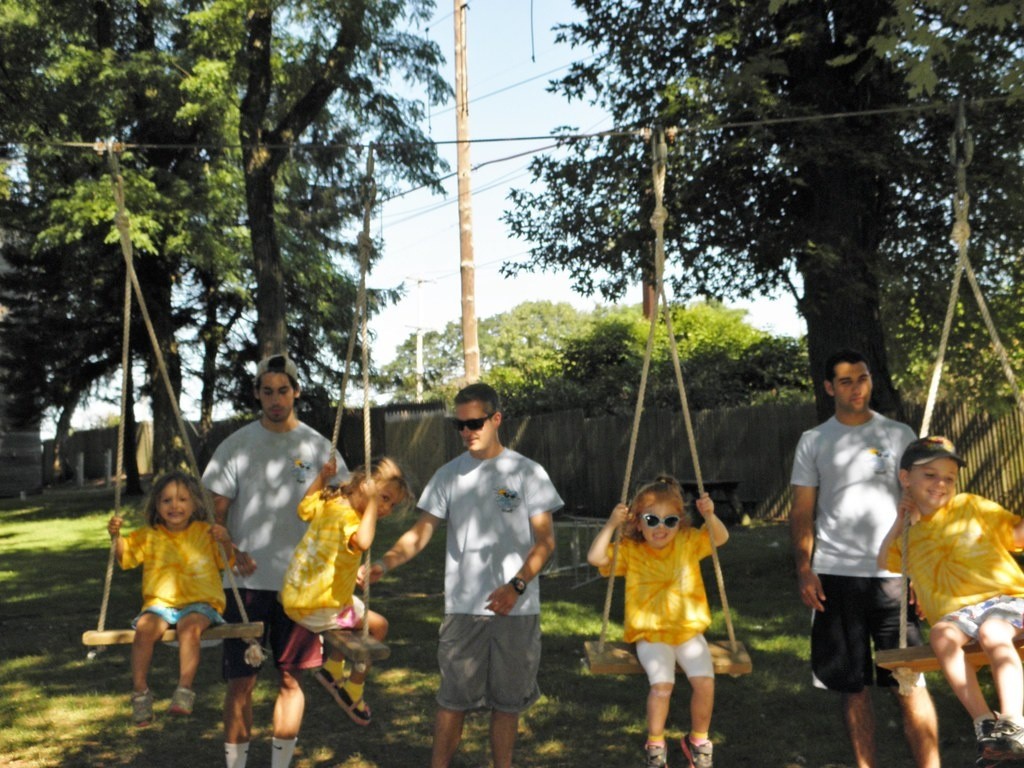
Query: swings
581,161,753,679
81,152,265,646
320,174,391,664
874,192,1024,697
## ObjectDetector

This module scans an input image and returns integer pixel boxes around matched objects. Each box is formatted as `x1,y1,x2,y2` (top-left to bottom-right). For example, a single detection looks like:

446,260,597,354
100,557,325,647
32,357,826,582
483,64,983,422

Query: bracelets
376,559,387,575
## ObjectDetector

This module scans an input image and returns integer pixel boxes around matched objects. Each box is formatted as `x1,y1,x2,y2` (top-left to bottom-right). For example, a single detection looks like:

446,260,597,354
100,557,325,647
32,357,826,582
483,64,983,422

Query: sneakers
680,733,713,768
131,687,153,726
168,687,195,714
975,713,1024,768
645,740,668,768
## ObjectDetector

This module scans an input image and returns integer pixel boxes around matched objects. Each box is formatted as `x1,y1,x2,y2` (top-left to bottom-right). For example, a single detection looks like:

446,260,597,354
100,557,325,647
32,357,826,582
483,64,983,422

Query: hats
901,436,966,469
256,355,298,385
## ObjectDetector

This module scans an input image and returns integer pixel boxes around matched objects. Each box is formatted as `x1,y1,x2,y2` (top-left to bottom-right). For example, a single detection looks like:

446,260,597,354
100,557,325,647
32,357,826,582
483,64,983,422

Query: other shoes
316,667,347,697
335,687,371,726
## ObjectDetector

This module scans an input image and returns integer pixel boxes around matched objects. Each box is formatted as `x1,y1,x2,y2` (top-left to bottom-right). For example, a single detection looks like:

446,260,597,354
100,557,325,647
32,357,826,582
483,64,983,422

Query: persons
586,474,729,768
788,350,942,768
878,435,1024,768
279,450,416,725
201,355,351,768
356,382,564,768
109,469,235,728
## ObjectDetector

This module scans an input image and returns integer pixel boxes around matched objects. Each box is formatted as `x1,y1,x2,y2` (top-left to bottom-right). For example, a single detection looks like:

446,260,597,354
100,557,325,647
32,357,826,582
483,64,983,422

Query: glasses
454,412,494,430
638,514,681,529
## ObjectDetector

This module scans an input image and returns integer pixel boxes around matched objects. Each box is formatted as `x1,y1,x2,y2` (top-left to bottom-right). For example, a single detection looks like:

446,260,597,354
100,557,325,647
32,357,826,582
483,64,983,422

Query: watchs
509,576,526,595
